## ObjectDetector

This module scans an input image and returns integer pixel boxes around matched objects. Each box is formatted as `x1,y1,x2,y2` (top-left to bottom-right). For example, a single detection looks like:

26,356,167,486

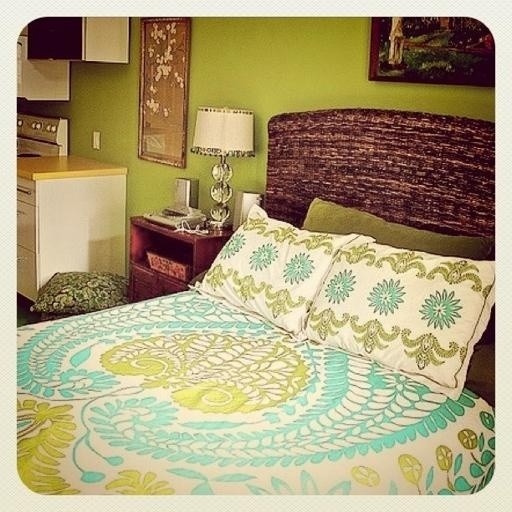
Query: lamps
191,107,256,228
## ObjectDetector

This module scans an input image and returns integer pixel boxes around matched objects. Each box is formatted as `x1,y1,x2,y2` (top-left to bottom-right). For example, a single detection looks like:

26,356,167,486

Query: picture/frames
366,13,495,88
136,19,186,169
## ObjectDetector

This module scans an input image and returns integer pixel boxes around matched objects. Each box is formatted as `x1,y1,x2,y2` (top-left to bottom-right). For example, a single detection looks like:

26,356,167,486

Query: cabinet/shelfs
26,17,129,64
17,174,128,303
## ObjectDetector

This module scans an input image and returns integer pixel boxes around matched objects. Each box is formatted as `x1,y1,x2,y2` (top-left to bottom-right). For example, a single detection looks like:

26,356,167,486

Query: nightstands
129,214,236,306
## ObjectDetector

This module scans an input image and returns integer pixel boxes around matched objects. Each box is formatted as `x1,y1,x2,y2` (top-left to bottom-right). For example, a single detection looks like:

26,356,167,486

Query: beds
17,107,495,496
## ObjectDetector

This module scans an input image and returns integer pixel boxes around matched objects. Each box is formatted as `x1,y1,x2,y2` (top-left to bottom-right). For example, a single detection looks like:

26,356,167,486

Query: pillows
30,271,129,322
188,204,361,342
303,197,494,401
299,234,495,401
